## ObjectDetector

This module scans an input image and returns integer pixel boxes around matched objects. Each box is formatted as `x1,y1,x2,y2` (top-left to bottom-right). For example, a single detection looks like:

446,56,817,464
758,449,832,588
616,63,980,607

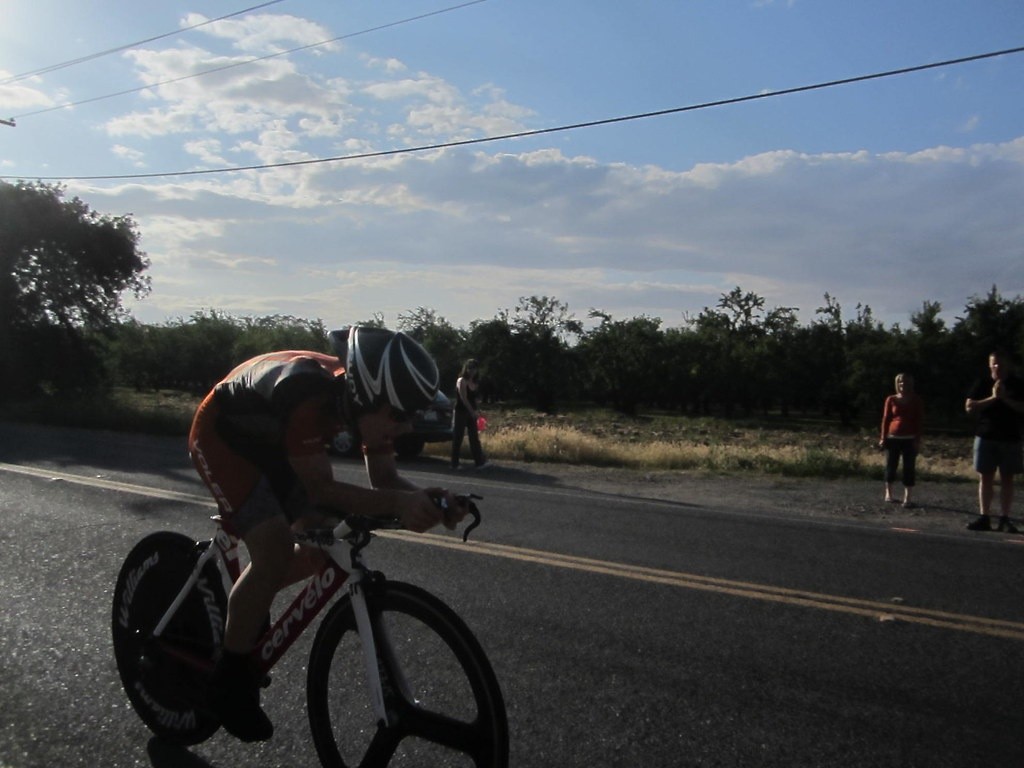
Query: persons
448,358,494,472
964,350,1024,532
878,373,924,506
187,327,470,742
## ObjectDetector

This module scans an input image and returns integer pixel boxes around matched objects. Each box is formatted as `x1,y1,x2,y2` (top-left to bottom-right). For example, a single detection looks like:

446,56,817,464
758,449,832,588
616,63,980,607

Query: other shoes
192,668,274,744
884,492,894,502
966,518,992,530
997,515,1018,533
901,501,912,508
474,462,493,471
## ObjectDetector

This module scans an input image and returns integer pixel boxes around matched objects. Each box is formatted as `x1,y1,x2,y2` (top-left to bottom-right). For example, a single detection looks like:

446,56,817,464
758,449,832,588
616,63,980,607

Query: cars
391,389,457,467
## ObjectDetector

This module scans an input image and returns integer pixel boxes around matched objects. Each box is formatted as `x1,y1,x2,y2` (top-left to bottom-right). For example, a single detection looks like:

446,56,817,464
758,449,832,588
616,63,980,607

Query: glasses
384,404,418,425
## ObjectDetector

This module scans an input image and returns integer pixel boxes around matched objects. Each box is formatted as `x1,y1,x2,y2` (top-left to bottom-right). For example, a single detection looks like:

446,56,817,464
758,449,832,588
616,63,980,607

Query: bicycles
107,479,509,768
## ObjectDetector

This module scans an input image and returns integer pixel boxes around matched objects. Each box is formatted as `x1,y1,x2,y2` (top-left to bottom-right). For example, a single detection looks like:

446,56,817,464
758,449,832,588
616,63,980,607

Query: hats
330,325,450,422
